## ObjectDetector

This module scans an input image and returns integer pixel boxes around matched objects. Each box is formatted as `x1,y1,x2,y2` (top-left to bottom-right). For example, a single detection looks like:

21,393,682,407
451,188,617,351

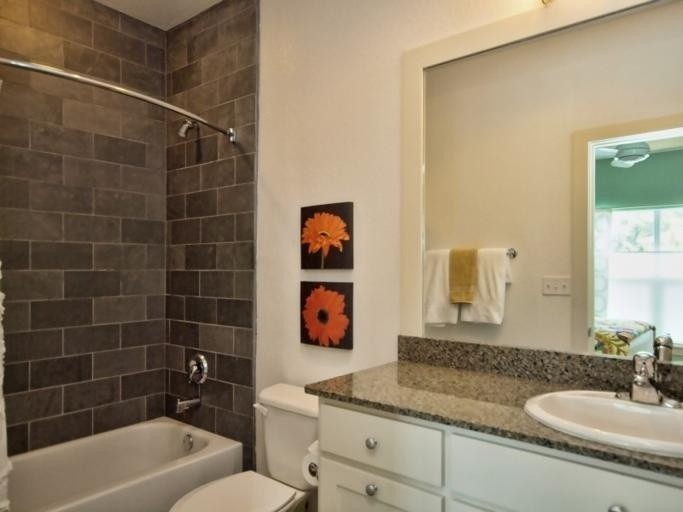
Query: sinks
524,388,683,458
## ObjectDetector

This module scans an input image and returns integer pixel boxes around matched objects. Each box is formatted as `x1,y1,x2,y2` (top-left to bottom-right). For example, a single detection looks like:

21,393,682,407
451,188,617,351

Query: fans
594,140,682,170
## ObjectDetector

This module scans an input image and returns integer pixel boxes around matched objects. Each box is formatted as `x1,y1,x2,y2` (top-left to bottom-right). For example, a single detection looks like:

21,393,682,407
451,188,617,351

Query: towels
459,245,514,325
423,249,459,327
449,249,475,304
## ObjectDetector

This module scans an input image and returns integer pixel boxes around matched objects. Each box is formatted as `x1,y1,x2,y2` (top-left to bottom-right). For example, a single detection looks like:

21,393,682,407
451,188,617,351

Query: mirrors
588,127,683,365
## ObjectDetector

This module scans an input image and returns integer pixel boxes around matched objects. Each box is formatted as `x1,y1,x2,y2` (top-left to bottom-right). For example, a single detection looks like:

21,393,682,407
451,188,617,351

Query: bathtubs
9,415,245,512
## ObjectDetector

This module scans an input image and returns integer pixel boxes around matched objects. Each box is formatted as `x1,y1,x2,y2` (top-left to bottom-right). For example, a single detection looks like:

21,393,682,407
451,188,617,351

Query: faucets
629,351,662,407
174,397,203,414
654,335,673,362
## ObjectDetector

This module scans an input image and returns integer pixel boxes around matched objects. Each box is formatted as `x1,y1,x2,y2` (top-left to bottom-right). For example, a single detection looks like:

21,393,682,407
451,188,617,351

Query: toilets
168,381,319,511
